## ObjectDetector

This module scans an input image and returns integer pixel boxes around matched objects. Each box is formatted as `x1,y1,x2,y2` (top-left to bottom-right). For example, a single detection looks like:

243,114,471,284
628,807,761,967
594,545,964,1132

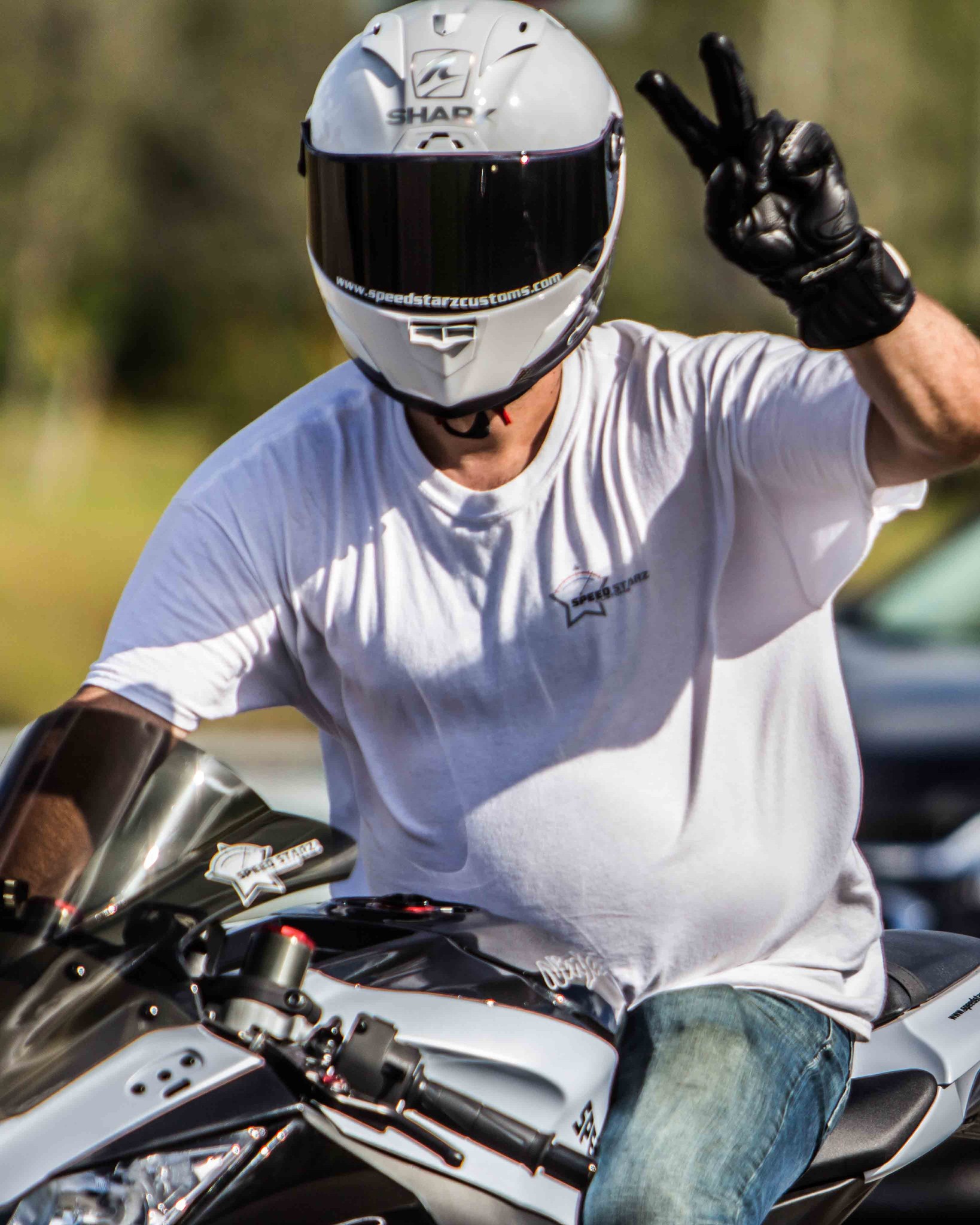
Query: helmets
292,0,631,426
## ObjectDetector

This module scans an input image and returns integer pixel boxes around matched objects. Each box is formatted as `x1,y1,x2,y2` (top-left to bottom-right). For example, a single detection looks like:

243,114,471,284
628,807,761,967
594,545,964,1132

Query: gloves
639,25,915,352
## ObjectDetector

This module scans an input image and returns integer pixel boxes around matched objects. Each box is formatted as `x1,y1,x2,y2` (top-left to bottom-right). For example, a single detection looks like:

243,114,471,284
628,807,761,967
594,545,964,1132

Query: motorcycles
1,707,979,1225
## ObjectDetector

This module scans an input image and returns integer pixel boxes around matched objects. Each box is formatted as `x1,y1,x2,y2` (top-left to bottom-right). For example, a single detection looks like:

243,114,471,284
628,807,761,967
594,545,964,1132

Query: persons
0,0,980,1225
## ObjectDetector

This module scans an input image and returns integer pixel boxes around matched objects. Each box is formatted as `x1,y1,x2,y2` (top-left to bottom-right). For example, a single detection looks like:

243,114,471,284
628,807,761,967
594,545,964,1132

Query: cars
822,503,980,935
